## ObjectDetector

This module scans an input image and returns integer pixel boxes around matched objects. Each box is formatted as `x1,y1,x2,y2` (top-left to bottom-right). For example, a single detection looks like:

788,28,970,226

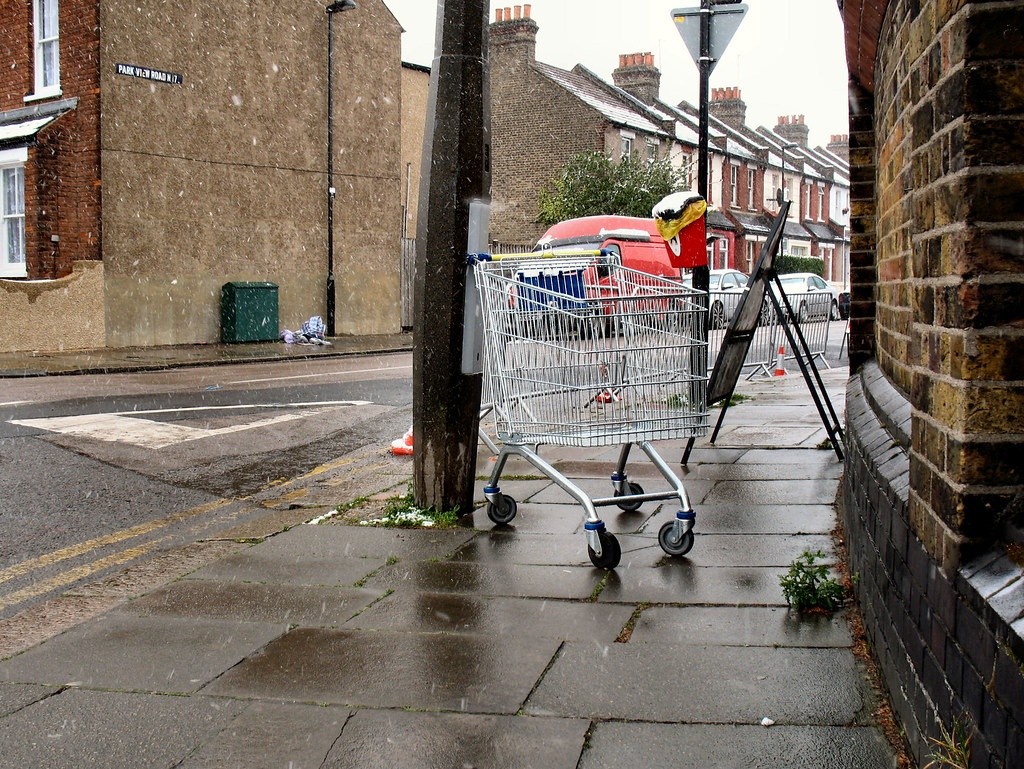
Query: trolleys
468,245,710,572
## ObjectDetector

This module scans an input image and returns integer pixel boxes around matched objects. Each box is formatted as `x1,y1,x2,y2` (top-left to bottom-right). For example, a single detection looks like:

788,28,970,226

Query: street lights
323,2,355,336
779,142,800,261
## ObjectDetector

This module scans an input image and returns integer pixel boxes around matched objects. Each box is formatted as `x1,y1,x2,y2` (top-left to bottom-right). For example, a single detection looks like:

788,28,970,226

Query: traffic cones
389,423,414,455
594,365,624,403
770,345,785,378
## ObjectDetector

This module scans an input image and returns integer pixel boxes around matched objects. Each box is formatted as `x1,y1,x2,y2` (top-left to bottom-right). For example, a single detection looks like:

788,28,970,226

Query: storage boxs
651,192,710,268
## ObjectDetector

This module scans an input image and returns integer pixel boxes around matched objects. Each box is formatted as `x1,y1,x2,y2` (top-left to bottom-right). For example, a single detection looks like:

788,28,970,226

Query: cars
769,273,839,324
681,268,771,329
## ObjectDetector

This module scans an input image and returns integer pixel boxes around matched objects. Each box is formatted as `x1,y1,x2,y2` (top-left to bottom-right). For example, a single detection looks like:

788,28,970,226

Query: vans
506,213,686,340
